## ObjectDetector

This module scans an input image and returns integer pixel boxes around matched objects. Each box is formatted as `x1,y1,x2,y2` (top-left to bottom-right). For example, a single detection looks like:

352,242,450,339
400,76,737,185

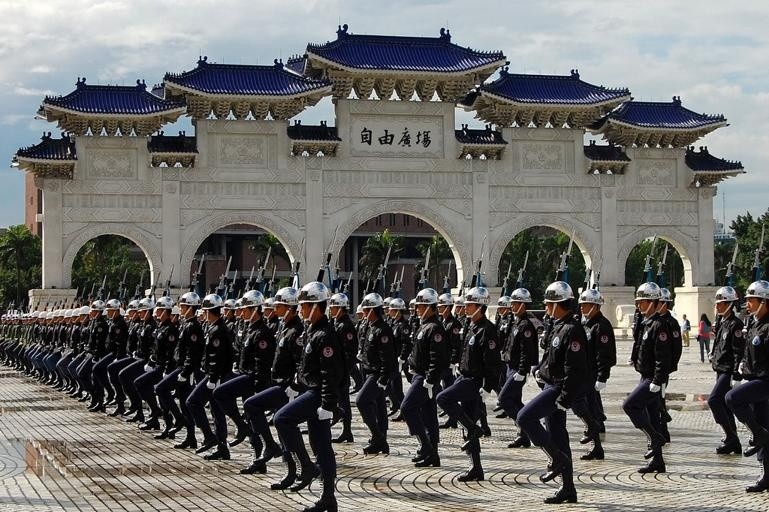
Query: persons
118,298,160,431
170,306,180,324
436,294,463,429
187,294,233,461
681,314,691,347
213,290,276,474
388,298,411,421
437,286,497,481
725,280,769,492
156,292,205,449
93,299,127,417
224,299,235,333
622,282,672,473
494,296,514,417
327,293,356,443
383,298,393,404
273,282,342,512
244,287,304,490
707,287,745,454
454,296,466,328
699,313,713,363
571,289,617,461
356,293,393,454
479,402,491,437
655,288,682,443
400,288,447,467
124,308,131,321
497,288,531,448
233,299,242,336
540,312,556,348
350,303,368,395
263,298,279,333
0,306,91,402
79,301,108,413
120,308,126,321
134,296,180,439
108,300,145,422
517,281,589,504
402,298,416,385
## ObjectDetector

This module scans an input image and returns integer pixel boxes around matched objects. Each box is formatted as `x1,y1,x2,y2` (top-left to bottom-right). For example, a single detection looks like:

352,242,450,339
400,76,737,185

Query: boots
507,420,532,448
542,464,578,504
414,441,441,467
363,422,390,456
579,412,602,444
172,427,199,450
579,433,604,460
103,383,115,406
715,416,742,455
637,446,666,473
152,414,174,440
438,410,447,417
411,429,433,461
456,447,485,482
167,405,186,434
253,426,282,464
745,459,769,494
271,449,296,490
331,419,354,442
661,401,672,424
144,400,163,424
390,407,407,422
303,477,338,511
388,407,399,417
121,385,141,416
230,412,253,447
0,349,91,403
641,424,667,460
202,436,231,462
108,401,126,417
449,409,485,451
138,406,159,431
108,381,128,407
89,385,106,412
367,427,387,444
239,445,268,475
126,403,146,423
289,447,319,492
197,423,218,454
538,440,572,483
744,416,761,457
496,409,511,418
152,418,176,440
439,415,458,429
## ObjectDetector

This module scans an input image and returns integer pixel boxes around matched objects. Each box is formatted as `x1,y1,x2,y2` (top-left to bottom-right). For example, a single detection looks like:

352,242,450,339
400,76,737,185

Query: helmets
201,294,225,322
179,291,200,319
64,309,74,325
154,296,174,320
328,292,350,318
136,297,155,321
28,314,32,324
71,308,80,323
633,281,665,315
239,289,266,324
52,310,59,322
414,287,440,320
408,298,416,317
272,286,299,320
657,287,674,315
436,293,455,316
387,297,406,319
79,305,90,323
232,297,244,319
543,281,575,319
126,300,140,320
91,300,106,320
45,312,52,325
361,292,385,322
509,287,533,315
463,286,490,319
58,309,67,323
382,297,394,315
0,313,28,325
455,295,465,315
496,295,512,316
222,299,236,317
262,297,274,320
297,281,331,324
744,279,768,319
356,303,365,314
578,288,605,318
170,306,179,322
37,311,47,325
106,299,121,321
32,311,40,323
714,286,739,316
